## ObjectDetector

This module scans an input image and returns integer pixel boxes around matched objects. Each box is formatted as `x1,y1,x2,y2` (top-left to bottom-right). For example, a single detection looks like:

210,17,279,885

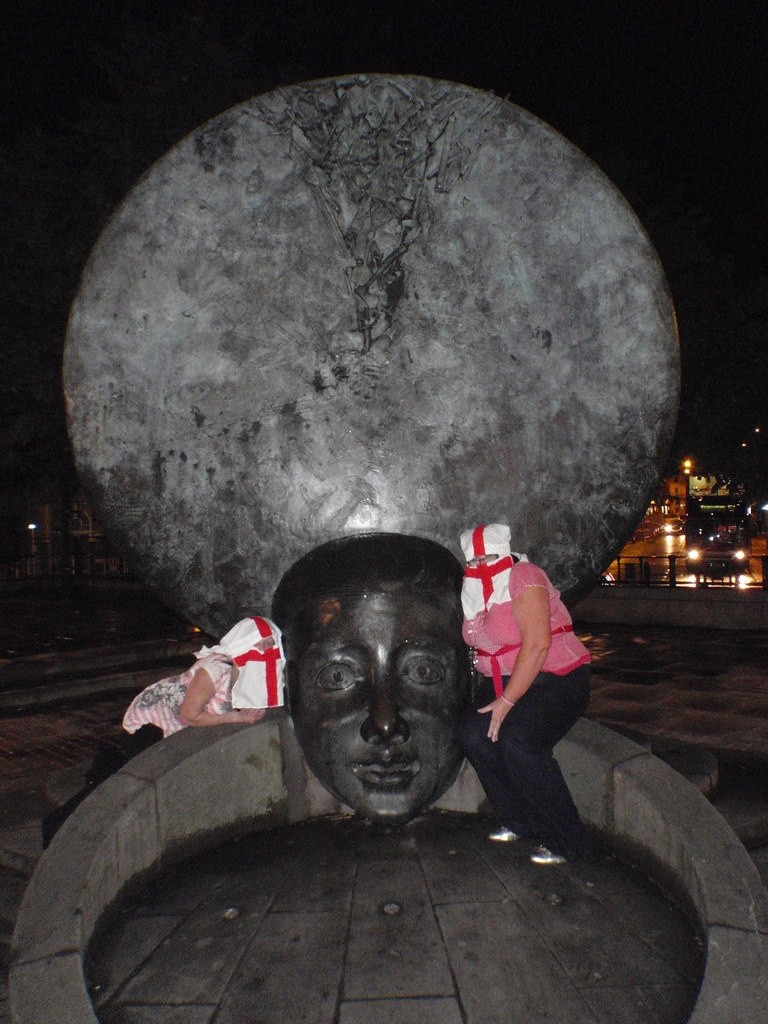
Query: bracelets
500,694,515,707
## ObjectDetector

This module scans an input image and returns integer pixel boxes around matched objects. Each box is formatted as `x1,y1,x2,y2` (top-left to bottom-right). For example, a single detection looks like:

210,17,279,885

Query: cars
601,573,616,586
664,520,685,533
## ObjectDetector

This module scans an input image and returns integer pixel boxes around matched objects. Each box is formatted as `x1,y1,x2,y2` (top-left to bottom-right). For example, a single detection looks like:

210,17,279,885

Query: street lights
28,524,37,573
684,460,692,494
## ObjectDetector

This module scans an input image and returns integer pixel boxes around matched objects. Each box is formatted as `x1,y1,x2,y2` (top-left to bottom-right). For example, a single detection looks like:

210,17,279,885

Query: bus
685,493,752,569
632,511,665,542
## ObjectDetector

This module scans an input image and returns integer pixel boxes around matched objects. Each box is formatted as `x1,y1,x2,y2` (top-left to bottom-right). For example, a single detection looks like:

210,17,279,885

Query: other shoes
531,844,565,864
489,826,516,841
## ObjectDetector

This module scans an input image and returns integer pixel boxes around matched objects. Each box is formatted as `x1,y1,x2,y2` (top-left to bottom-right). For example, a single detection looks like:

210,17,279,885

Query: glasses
467,554,497,567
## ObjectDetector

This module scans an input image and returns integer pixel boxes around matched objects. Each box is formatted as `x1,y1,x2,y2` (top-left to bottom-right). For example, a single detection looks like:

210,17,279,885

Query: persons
272,531,476,831
41,614,286,852
451,523,593,867
